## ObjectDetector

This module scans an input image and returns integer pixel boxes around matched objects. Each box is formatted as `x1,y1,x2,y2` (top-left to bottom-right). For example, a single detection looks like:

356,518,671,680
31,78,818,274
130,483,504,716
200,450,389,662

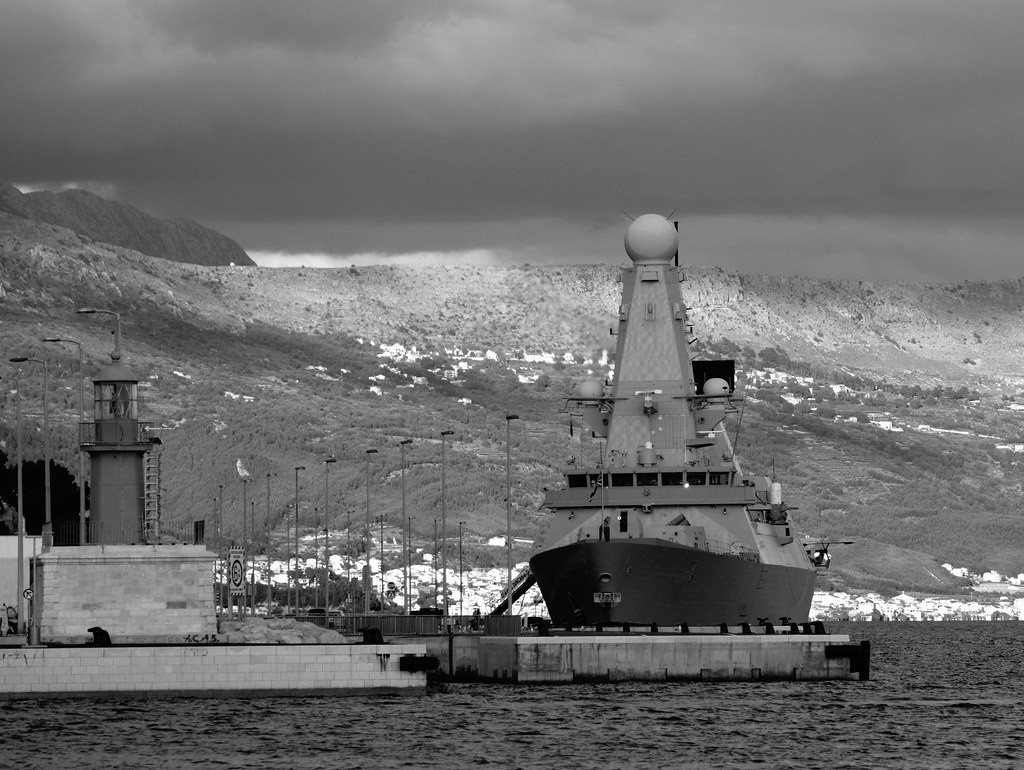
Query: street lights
267,473,277,617
213,485,225,612
366,449,378,615
326,458,337,617
440,430,454,615
295,466,306,617
459,522,467,616
76,308,121,351
287,505,294,614
9,357,51,523
401,439,413,616
506,415,520,616
347,511,442,612
315,508,323,608
42,337,86,546
251,502,257,613
243,479,252,618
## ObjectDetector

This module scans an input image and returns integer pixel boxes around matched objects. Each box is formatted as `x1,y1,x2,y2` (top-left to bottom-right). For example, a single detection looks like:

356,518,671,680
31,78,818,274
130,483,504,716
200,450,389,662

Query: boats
530,208,854,627
